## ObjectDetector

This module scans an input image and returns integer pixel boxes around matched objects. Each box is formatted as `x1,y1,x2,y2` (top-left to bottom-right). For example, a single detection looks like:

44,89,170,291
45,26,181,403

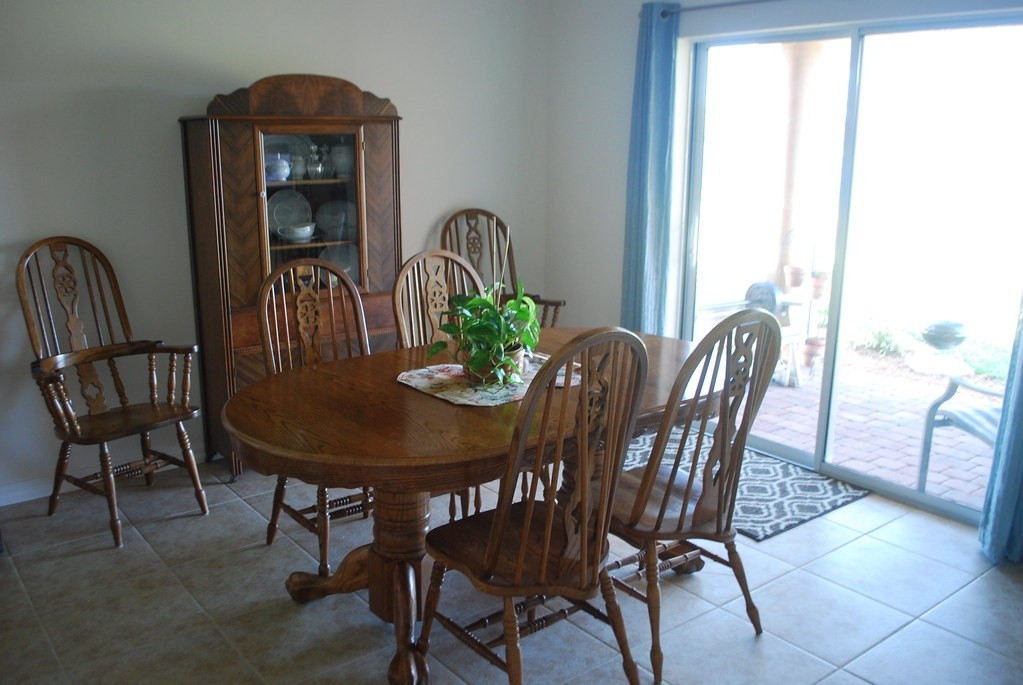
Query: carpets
621,423,869,542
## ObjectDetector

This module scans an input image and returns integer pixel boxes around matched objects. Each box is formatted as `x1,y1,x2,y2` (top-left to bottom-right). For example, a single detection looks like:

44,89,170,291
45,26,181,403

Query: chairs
15,236,209,550
261,257,375,580
917,375,1007,507
390,248,486,351
567,308,782,684
439,207,567,340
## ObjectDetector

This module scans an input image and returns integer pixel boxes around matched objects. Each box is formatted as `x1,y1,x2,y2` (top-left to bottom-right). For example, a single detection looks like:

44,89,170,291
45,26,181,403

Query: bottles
307,143,335,179
331,137,354,178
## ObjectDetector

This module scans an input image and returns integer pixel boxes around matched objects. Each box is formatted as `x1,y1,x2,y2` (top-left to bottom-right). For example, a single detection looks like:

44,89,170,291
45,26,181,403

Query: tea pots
265,150,311,181
277,223,316,243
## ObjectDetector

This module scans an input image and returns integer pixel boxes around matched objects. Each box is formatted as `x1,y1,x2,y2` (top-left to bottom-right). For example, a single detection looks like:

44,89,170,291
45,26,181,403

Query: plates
263,134,313,169
267,189,312,237
316,200,357,240
318,243,359,288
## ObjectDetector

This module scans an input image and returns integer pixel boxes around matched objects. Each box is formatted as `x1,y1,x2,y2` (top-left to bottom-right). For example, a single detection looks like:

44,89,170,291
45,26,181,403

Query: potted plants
424,276,543,395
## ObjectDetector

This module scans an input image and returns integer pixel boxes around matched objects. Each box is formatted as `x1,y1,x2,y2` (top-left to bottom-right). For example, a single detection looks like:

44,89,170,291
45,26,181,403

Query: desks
220,323,740,684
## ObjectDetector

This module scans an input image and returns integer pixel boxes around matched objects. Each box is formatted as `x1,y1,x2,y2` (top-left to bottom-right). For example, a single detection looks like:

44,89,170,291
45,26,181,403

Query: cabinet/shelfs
178,72,404,483
418,327,651,684
